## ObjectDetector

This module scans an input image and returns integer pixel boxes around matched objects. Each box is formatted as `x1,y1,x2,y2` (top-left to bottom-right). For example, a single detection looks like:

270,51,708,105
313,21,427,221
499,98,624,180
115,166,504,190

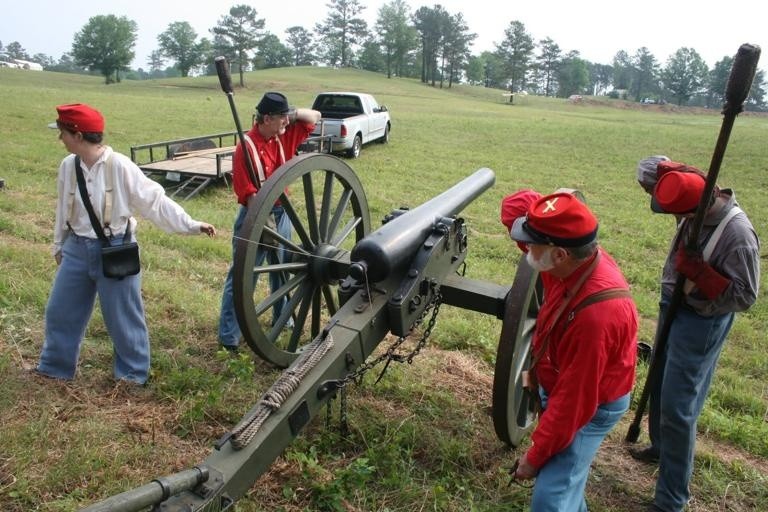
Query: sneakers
634,443,661,464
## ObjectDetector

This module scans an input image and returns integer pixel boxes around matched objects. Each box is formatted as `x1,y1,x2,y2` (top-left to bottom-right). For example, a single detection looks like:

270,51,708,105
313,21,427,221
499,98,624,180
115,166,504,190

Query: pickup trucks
305,91,393,160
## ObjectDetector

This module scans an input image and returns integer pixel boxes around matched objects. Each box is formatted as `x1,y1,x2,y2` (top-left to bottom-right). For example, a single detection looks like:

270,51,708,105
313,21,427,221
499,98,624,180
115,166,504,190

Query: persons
31,104,217,388
501,190,639,512
637,155,760,511
218,92,322,351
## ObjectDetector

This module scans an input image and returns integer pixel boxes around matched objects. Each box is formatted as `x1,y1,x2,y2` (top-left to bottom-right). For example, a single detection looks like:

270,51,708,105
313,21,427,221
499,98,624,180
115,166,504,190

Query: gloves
657,160,705,179
669,238,732,303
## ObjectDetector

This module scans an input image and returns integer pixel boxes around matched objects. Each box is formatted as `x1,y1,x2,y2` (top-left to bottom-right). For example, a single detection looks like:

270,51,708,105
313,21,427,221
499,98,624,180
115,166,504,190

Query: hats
510,192,599,248
46,104,104,135
650,170,707,215
256,92,297,116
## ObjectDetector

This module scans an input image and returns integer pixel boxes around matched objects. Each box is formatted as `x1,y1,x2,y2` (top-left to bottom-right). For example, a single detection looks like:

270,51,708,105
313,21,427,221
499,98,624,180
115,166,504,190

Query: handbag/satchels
101,241,141,279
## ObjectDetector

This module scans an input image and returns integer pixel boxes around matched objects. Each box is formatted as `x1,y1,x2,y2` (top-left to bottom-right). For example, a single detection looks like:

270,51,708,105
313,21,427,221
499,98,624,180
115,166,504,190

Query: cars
639,97,655,105
